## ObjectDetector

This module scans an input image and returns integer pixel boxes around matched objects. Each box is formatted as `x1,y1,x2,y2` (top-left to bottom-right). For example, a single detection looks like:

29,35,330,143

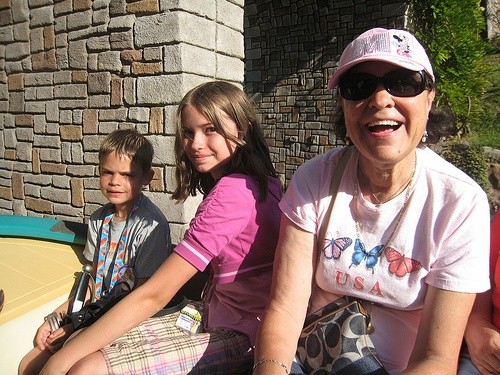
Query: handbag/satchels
296,296,392,375
70,282,132,329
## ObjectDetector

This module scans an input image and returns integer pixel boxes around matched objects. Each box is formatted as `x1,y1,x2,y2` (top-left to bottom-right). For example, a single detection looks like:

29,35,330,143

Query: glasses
338,71,434,100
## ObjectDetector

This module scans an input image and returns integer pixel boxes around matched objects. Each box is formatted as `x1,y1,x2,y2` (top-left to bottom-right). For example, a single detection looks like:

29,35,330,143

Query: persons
18,128,169,375
252,27,492,375
39,81,283,375
457,208,500,375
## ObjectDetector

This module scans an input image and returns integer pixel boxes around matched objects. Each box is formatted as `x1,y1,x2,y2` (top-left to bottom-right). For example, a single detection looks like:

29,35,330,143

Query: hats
327,27,434,90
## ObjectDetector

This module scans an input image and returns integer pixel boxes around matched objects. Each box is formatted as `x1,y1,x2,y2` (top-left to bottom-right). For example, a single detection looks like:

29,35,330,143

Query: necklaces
358,167,413,206
351,174,409,256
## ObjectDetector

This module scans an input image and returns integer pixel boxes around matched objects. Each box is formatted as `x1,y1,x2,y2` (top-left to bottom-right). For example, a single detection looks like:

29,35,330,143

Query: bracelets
253,358,290,373
44,311,60,334
